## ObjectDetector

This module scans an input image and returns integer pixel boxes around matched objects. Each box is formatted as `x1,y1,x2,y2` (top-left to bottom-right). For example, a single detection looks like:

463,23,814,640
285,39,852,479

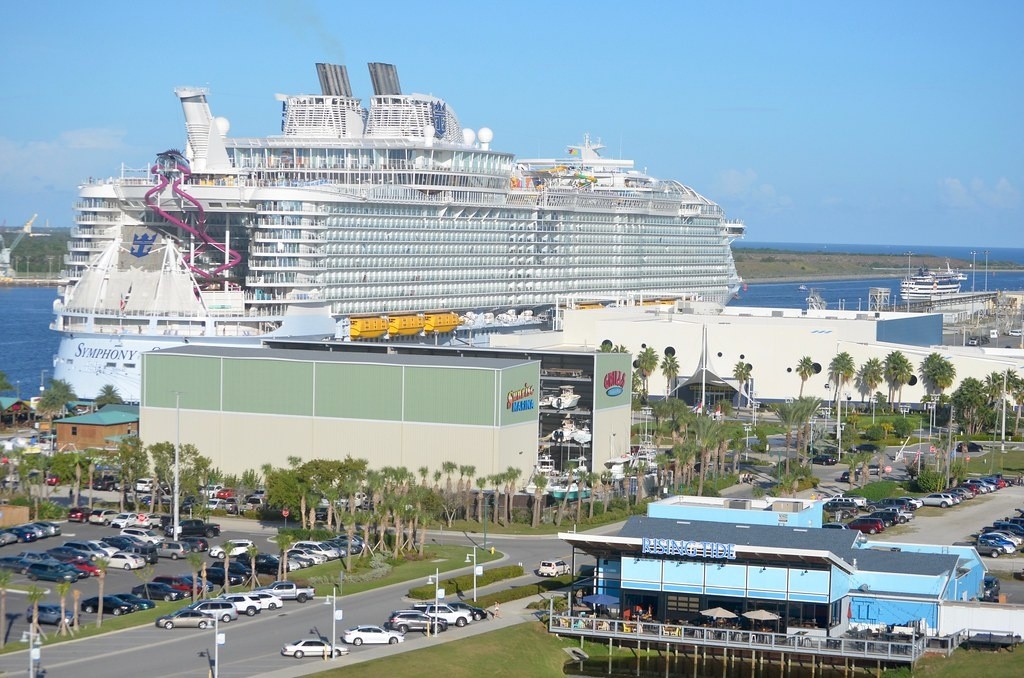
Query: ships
49,61,744,403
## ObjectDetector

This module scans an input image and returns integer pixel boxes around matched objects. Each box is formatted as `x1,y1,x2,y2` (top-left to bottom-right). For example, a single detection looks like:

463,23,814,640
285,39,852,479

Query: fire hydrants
490,545,496,555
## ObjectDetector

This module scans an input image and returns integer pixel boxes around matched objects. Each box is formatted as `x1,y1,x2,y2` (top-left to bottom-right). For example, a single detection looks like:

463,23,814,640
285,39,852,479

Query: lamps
763,559,766,570
721,561,724,567
604,560,608,565
805,567,808,573
679,558,682,564
637,555,640,561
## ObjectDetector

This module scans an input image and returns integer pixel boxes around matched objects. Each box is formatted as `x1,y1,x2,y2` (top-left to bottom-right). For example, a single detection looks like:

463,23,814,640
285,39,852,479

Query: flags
120,294,124,312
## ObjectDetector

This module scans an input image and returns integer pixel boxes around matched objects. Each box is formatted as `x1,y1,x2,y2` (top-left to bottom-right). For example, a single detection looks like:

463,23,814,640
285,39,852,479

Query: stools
789,637,812,647
694,630,770,644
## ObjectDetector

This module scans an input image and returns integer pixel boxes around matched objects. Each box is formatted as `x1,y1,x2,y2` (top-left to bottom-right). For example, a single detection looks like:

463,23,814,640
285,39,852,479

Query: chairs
560,615,679,636
826,629,908,655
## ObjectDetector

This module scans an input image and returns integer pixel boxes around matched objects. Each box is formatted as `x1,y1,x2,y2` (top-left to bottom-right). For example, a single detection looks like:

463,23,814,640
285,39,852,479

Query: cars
938,474,1017,503
823,494,867,508
840,472,859,483
156,608,215,630
955,442,983,453
343,625,405,646
952,508,1024,559
968,336,990,347
855,464,885,475
822,522,850,530
24,560,78,584
538,559,571,578
859,496,924,528
849,444,880,455
78,594,135,616
809,454,838,467
1,455,372,629
280,638,350,659
918,493,956,509
990,330,1000,339
186,600,239,623
447,602,487,622
845,518,885,535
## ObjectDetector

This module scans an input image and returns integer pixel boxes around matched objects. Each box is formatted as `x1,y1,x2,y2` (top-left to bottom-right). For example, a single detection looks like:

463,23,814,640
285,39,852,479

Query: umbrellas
700,608,738,618
583,594,620,616
742,610,784,629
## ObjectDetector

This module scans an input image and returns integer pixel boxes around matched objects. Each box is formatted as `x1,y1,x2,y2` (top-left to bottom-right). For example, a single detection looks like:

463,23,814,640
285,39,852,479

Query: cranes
0,212,40,281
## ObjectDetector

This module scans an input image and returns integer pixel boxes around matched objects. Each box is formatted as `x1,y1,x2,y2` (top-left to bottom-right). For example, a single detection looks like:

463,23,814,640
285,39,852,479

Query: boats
423,313,466,335
658,300,676,306
798,285,808,293
573,303,605,310
897,260,969,304
601,405,666,488
346,316,390,343
636,301,657,307
532,383,598,502
386,314,426,338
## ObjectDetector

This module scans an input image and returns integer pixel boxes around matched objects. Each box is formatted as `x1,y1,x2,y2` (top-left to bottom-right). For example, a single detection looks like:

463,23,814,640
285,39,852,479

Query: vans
1009,329,1024,337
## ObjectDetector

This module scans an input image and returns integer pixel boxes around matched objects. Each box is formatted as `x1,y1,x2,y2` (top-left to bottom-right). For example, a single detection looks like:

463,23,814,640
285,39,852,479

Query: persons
810,494,822,500
646,605,653,628
493,600,500,619
708,618,738,626
589,607,612,629
577,589,582,607
627,614,642,632
636,605,643,615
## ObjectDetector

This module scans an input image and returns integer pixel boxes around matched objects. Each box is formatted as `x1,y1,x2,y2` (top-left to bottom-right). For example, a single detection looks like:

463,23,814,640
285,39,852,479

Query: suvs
388,609,448,635
411,601,473,628
822,501,860,521
981,575,1001,602
26,603,80,626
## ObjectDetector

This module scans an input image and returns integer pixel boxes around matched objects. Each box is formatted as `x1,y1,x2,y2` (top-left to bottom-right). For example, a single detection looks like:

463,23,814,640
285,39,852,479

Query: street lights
324,587,337,659
983,250,993,311
484,491,495,552
199,614,220,678
465,546,478,603
742,423,754,461
18,623,43,678
969,249,980,312
902,250,915,313
424,568,439,637
